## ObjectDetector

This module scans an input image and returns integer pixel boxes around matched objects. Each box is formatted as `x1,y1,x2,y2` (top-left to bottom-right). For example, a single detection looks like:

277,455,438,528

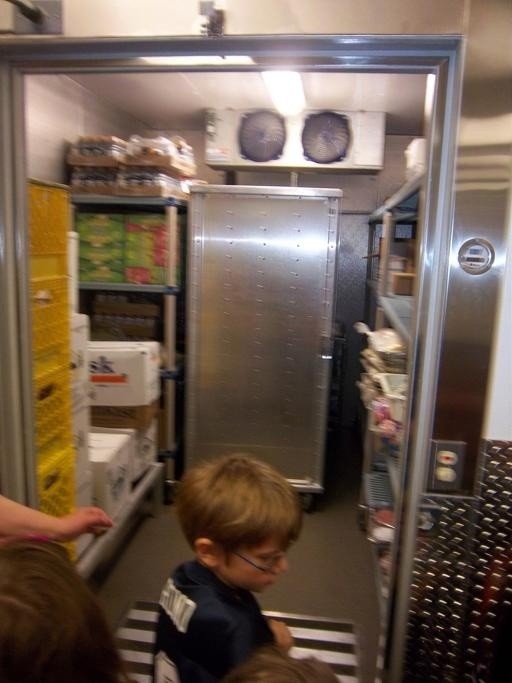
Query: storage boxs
67,207,183,517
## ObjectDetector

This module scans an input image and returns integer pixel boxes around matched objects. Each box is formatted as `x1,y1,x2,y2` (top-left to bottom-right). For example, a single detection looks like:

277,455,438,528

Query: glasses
228,545,280,573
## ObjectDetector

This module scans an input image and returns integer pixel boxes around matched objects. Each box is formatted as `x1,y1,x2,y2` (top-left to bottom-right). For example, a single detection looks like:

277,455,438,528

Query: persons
0,494,118,544
0,532,138,681
223,643,342,682
148,452,305,683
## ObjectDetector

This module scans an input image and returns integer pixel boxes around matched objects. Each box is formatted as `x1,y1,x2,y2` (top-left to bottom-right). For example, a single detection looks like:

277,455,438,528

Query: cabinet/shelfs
352,170,423,621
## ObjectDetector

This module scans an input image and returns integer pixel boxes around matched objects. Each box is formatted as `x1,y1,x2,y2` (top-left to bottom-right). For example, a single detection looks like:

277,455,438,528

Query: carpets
112,600,358,683
